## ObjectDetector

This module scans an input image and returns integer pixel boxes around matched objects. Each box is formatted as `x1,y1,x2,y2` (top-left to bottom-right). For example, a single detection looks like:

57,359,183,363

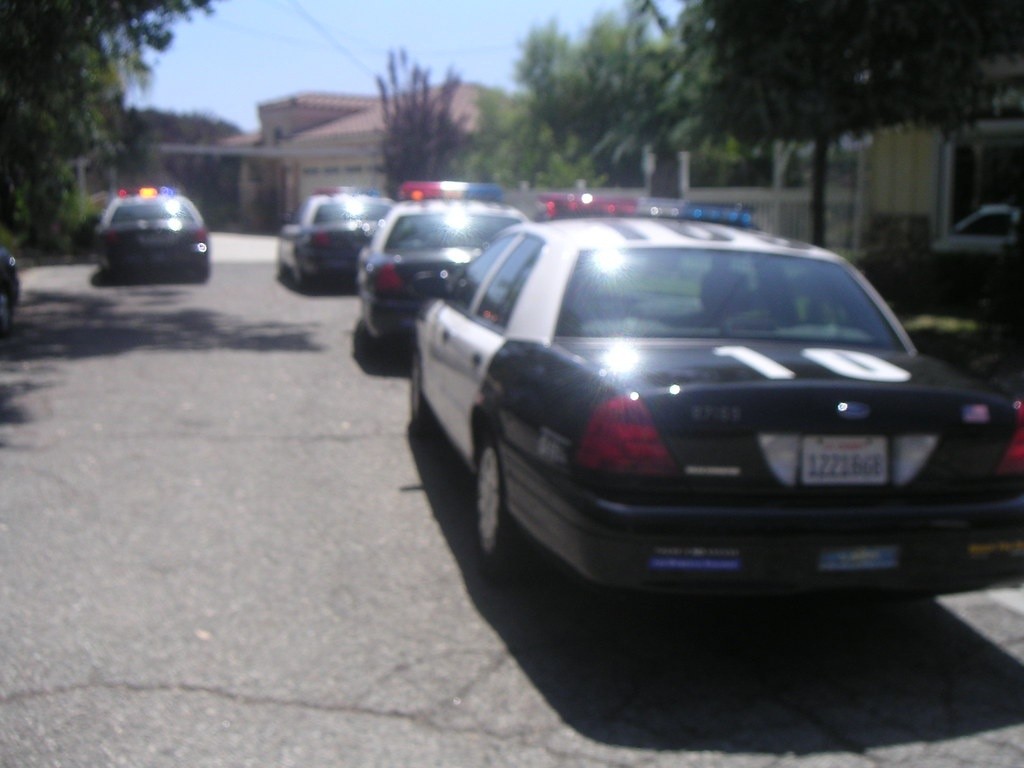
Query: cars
95,184,212,279
278,188,401,288
0,247,21,334
409,190,1023,594
352,180,535,332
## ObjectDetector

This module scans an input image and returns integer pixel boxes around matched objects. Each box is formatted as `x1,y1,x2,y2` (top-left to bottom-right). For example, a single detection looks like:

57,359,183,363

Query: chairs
692,268,765,331
